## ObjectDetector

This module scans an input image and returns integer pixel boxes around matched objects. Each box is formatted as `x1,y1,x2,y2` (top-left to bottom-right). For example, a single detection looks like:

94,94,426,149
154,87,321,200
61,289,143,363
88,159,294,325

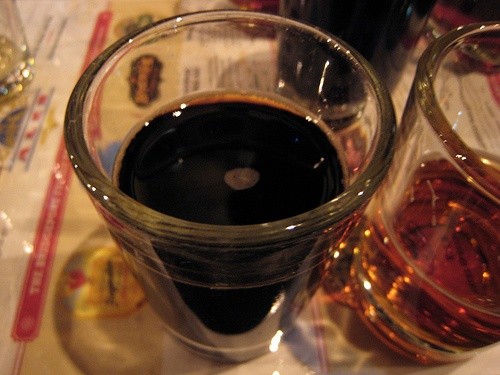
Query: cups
0,1,38,102
270,0,440,133
343,22,500,369
59,7,397,364
